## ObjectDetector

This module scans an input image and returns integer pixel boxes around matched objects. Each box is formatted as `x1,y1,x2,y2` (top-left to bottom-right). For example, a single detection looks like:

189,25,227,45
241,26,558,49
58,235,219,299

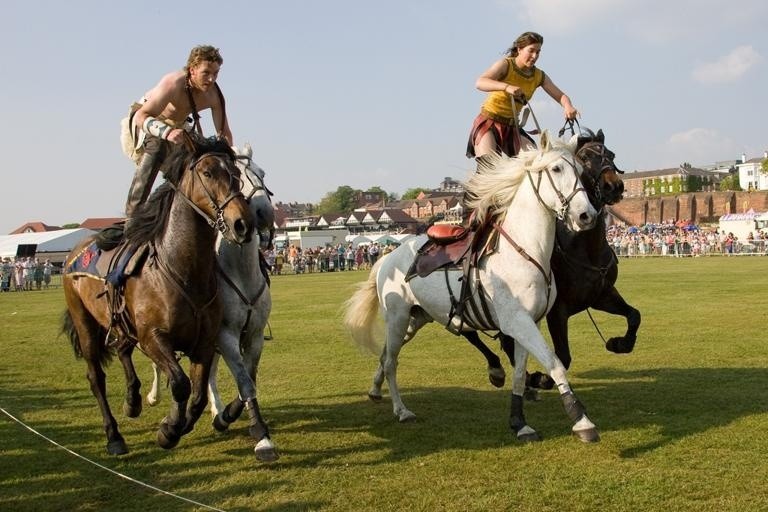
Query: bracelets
142,115,173,141
504,84,510,92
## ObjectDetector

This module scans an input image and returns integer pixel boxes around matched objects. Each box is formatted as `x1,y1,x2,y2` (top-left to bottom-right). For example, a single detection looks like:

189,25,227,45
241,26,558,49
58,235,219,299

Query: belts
481,108,517,126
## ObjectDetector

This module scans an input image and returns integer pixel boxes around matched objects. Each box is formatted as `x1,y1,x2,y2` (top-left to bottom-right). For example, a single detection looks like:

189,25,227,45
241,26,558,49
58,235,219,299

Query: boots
463,154,496,222
126,154,168,232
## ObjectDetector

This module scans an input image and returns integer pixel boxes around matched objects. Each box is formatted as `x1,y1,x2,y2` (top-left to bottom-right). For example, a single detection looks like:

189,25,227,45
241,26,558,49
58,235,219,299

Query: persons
604,219,767,257
0,252,69,292
462,31,578,229
266,239,395,276
121,45,234,241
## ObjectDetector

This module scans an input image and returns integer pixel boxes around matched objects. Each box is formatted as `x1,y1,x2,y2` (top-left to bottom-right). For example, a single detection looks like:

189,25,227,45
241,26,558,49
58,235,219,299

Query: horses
458,129,641,444
337,125,602,443
56,126,254,457
144,138,279,462
